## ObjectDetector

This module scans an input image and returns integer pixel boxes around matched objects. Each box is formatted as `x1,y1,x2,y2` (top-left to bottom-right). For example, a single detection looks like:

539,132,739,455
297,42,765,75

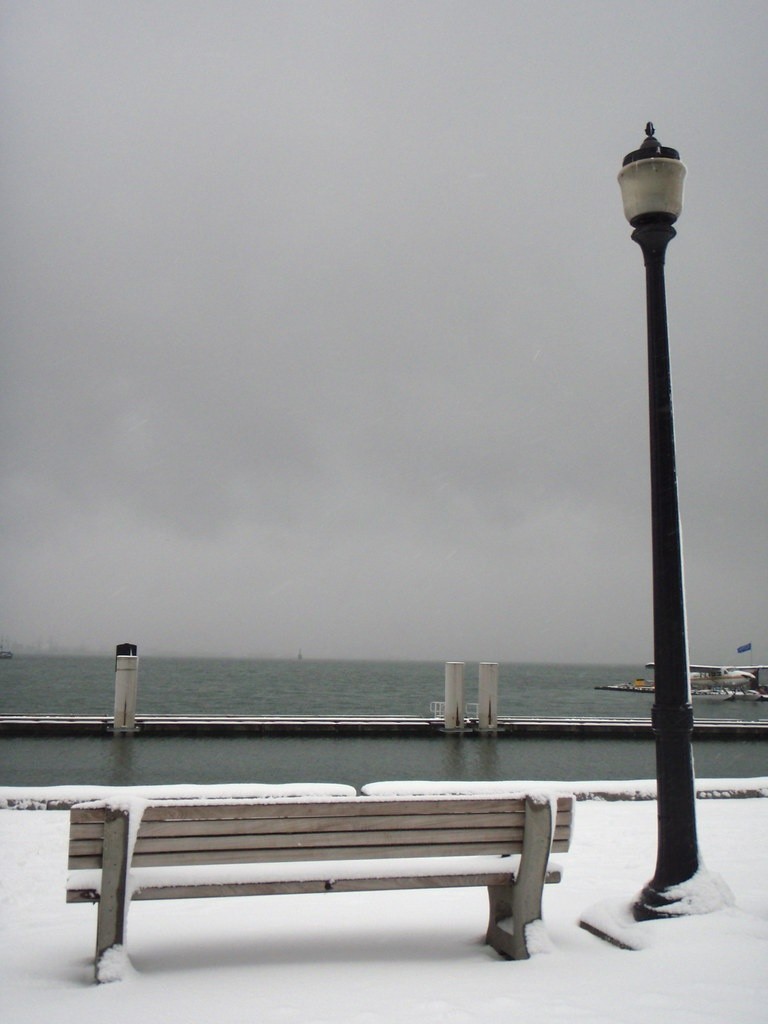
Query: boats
595,665,768,700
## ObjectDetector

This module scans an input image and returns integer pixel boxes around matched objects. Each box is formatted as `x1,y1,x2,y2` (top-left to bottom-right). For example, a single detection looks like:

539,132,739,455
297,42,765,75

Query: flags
737,643,751,653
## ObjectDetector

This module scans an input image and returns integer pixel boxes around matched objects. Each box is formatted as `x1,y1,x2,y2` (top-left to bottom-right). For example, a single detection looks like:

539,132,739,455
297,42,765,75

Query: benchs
64,791,576,986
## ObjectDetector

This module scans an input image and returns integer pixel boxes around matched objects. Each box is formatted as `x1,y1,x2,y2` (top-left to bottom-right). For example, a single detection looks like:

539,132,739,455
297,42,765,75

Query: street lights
615,118,731,928
